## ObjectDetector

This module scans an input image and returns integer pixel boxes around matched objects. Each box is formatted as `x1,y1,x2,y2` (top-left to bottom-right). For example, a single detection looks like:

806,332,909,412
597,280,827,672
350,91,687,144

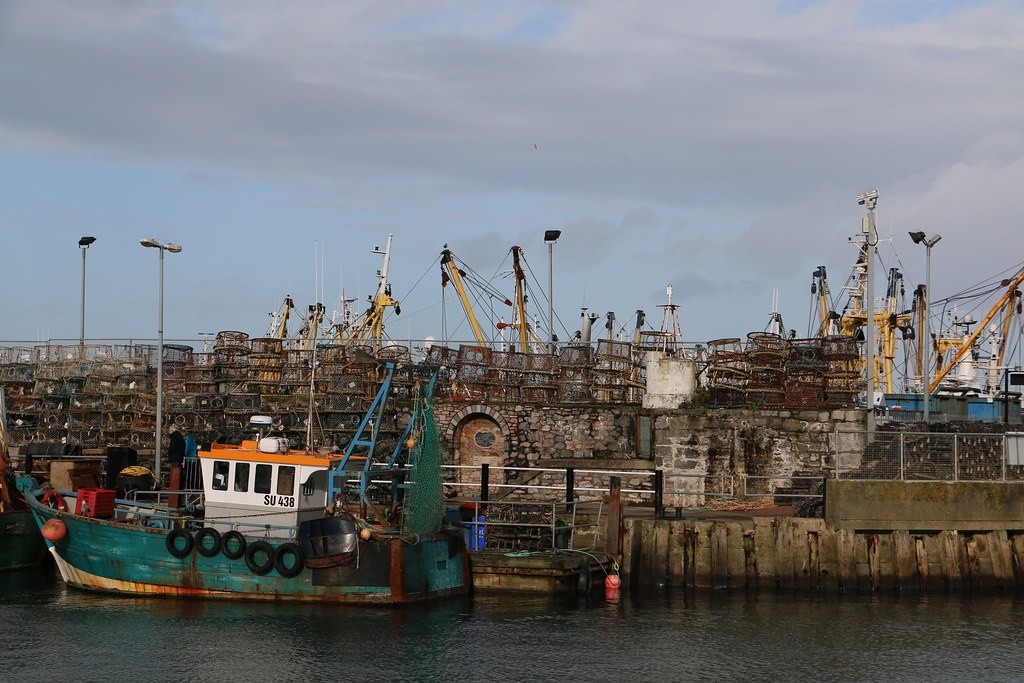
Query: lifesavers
195,527,222,557
166,527,194,559
220,530,247,560
244,541,274,575
274,543,304,578
43,490,65,511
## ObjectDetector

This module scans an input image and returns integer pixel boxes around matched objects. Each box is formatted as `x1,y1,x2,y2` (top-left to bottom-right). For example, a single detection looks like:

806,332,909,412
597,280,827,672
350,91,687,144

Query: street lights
542,229,562,353
78,236,96,373
140,237,182,482
909,232,942,425
850,236,875,444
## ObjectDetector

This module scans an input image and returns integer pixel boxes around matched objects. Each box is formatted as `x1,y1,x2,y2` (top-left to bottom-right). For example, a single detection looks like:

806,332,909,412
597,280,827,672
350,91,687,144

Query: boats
0,415,52,573
15,315,472,605
